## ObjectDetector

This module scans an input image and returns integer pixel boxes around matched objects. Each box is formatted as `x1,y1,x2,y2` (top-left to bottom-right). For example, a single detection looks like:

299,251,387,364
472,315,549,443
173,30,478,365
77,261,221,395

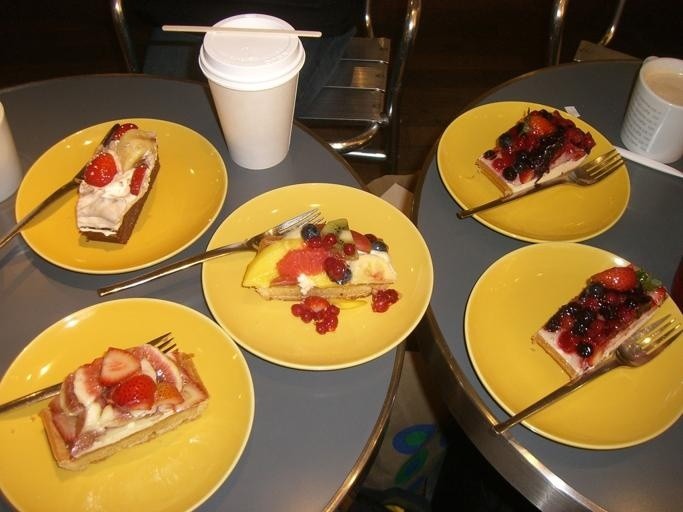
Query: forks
0,123,123,249
97,208,328,297
491,313,682,436
455,149,626,220
0,332,180,415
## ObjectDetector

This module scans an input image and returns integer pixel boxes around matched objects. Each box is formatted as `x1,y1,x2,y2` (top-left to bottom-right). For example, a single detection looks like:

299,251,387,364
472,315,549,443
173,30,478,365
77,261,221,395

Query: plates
464,241,683,450
0,296,256,512
201,182,435,373
436,100,632,244
15,116,230,275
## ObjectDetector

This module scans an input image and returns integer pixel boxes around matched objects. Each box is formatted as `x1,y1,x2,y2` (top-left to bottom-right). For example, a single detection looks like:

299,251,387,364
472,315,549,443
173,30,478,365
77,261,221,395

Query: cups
197,13,307,171
619,56,683,164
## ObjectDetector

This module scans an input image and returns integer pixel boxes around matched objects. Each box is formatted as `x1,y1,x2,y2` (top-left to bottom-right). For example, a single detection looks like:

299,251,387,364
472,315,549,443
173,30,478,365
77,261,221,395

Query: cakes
243,218,402,332
475,108,596,198
536,265,670,378
76,124,161,243
38,344,209,472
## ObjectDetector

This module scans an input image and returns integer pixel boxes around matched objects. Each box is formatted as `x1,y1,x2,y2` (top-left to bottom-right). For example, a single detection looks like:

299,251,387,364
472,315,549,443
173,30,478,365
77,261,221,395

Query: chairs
109,1,426,175
542,1,642,68
